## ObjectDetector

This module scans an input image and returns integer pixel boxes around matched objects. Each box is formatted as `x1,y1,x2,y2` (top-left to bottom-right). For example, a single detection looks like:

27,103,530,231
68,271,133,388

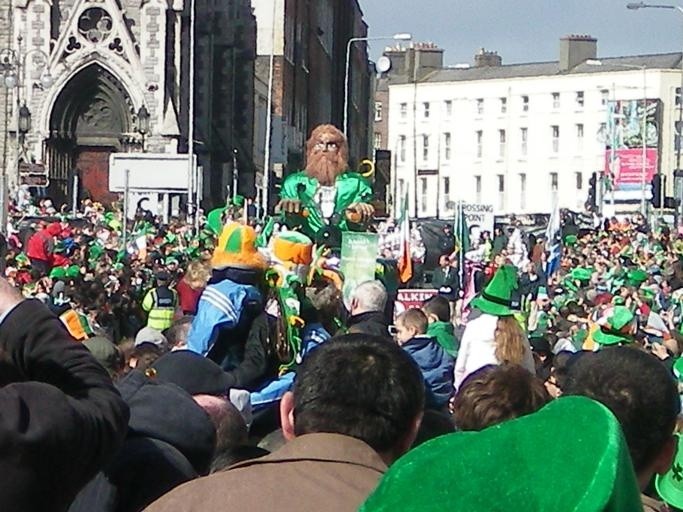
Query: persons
605,99,660,147
1,180,682,512
276,124,377,250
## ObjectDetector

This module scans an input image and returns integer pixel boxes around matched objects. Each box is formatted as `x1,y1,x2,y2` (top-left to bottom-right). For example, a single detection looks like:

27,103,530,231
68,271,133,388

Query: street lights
585,58,647,215
344,33,412,140
1,48,53,239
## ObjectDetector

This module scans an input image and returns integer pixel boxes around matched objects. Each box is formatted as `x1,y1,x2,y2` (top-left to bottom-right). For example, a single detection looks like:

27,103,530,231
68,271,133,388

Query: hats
359,395,642,512
158,272,167,280
591,306,635,345
80,337,125,377
472,265,523,315
